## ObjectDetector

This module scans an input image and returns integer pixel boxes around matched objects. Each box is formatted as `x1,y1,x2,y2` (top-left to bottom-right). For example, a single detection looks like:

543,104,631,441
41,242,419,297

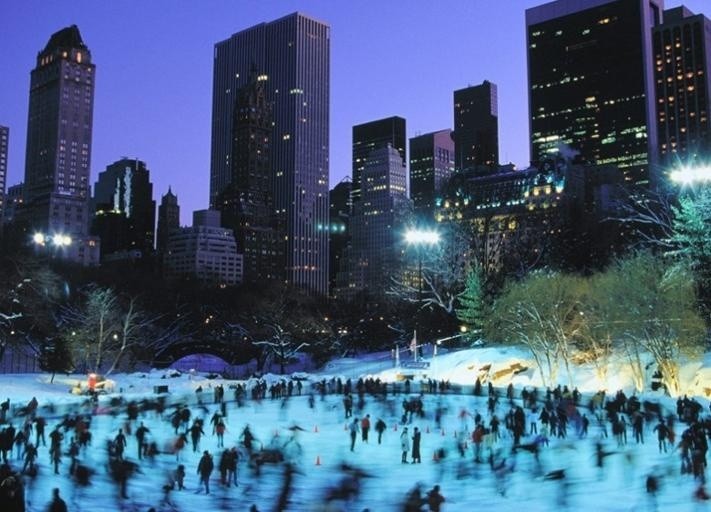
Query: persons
1,372,711,512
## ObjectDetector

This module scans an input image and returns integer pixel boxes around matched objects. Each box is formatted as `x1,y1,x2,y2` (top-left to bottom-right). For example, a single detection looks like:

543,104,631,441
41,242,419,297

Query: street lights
404,228,440,357
33,232,72,295
